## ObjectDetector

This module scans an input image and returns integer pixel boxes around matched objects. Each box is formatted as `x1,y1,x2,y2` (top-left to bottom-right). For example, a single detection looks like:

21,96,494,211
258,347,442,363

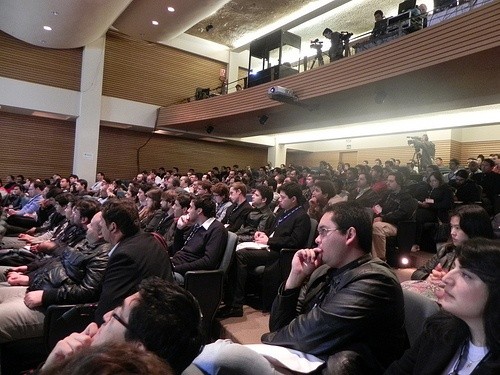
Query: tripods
310,48,330,70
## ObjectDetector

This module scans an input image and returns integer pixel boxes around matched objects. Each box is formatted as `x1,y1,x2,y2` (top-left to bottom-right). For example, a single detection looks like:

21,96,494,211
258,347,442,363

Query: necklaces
466,355,480,368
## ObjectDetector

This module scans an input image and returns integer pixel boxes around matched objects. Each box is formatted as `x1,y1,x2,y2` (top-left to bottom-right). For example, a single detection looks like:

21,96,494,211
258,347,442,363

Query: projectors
266,86,293,97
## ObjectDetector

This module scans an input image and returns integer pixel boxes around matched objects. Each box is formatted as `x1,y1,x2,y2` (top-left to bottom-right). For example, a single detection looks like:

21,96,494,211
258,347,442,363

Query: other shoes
411,244,420,252
214,305,244,318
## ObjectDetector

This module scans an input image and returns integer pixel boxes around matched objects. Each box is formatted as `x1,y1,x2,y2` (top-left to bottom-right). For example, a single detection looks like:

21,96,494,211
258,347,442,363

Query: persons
374,4,431,32
0,136,500,375
323,28,344,63
236,85,243,91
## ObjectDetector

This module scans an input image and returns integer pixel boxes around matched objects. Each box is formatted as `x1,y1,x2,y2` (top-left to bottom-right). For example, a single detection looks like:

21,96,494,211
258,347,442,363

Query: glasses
72,210,81,217
212,194,220,197
317,228,338,234
105,306,138,334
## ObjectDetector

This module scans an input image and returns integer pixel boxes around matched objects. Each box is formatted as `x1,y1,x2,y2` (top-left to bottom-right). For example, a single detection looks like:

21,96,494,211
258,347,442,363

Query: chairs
271,218,319,291
183,229,243,327
402,287,441,349
